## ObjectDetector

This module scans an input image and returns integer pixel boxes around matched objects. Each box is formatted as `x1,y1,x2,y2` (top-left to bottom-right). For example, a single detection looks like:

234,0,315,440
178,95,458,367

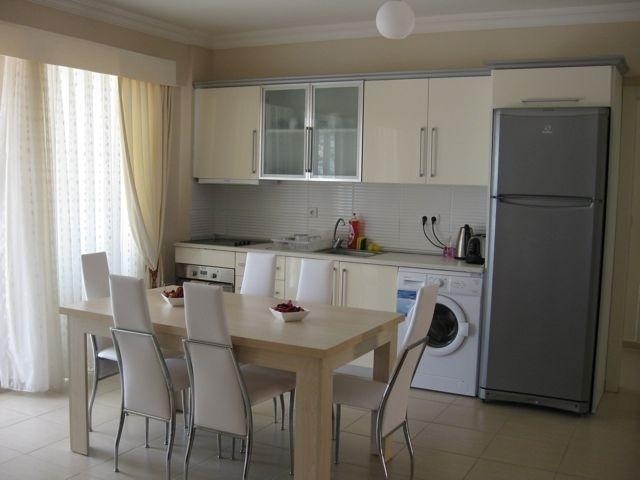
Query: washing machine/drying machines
394,265,483,398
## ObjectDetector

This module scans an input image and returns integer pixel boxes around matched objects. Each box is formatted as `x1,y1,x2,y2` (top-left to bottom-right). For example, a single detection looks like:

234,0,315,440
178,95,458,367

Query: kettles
456,223,474,260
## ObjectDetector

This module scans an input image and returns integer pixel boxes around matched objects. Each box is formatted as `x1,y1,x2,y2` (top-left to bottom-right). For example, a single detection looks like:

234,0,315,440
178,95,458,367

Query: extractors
195,178,280,186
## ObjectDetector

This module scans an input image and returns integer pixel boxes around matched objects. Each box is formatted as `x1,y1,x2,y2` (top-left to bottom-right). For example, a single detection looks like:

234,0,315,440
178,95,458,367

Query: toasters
466,234,486,264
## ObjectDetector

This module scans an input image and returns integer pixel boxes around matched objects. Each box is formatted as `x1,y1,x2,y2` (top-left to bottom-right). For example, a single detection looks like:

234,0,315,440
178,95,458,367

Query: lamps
375,0,417,41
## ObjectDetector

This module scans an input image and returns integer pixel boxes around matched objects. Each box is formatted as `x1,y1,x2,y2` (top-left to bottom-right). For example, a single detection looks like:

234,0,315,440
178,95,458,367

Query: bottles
347,213,361,249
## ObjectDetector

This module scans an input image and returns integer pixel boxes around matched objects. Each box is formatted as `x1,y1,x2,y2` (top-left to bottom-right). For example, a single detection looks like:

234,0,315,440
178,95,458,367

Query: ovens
174,264,235,284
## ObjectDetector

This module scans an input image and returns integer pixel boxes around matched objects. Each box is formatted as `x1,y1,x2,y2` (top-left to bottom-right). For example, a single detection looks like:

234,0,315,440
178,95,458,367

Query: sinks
314,246,385,258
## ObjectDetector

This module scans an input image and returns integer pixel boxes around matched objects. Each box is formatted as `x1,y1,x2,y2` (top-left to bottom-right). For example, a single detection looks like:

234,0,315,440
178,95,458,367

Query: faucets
332,215,345,250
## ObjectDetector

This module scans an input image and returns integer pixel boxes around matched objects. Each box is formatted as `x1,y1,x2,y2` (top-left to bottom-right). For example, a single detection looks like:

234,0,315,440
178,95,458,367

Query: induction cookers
180,234,273,246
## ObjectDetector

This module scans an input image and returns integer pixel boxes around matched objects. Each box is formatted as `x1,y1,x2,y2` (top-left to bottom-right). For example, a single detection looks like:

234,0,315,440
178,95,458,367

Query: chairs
112,277,190,480
179,284,295,479
331,284,439,479
80,251,178,432
276,259,350,439
211,250,278,454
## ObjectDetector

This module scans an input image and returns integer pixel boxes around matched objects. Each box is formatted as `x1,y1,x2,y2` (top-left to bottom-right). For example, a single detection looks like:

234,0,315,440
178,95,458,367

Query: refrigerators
478,107,609,413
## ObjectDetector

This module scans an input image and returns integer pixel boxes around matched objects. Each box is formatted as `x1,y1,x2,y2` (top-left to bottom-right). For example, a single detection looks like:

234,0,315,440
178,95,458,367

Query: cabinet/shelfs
259,77,365,181
192,82,261,187
285,257,398,366
358,75,491,184
234,253,285,298
